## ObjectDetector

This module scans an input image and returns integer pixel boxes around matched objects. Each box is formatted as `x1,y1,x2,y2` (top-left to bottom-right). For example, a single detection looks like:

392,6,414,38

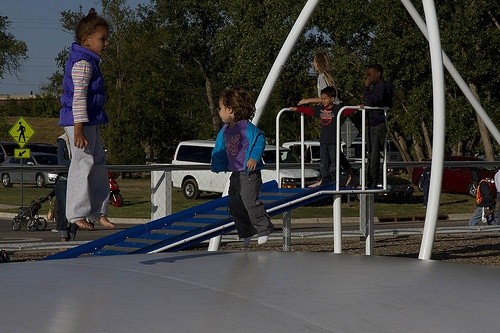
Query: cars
279,138,413,176
410,156,497,197
0,151,59,188
338,174,415,204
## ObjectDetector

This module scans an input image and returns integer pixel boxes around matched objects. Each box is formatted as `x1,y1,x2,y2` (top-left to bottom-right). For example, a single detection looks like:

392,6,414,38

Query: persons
53,133,78,241
58,8,116,229
469,168,498,233
313,52,344,182
211,86,274,248
289,87,364,188
417,167,431,209
364,64,392,189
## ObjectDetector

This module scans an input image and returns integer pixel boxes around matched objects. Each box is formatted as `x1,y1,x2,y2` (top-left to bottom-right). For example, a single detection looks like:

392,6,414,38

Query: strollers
11,189,56,232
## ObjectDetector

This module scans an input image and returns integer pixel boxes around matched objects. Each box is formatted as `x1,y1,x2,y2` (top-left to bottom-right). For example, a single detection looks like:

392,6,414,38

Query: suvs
0,142,58,164
171,140,322,200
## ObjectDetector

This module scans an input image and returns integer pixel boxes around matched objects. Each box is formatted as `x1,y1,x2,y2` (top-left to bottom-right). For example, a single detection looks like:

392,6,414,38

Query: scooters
107,171,123,207
